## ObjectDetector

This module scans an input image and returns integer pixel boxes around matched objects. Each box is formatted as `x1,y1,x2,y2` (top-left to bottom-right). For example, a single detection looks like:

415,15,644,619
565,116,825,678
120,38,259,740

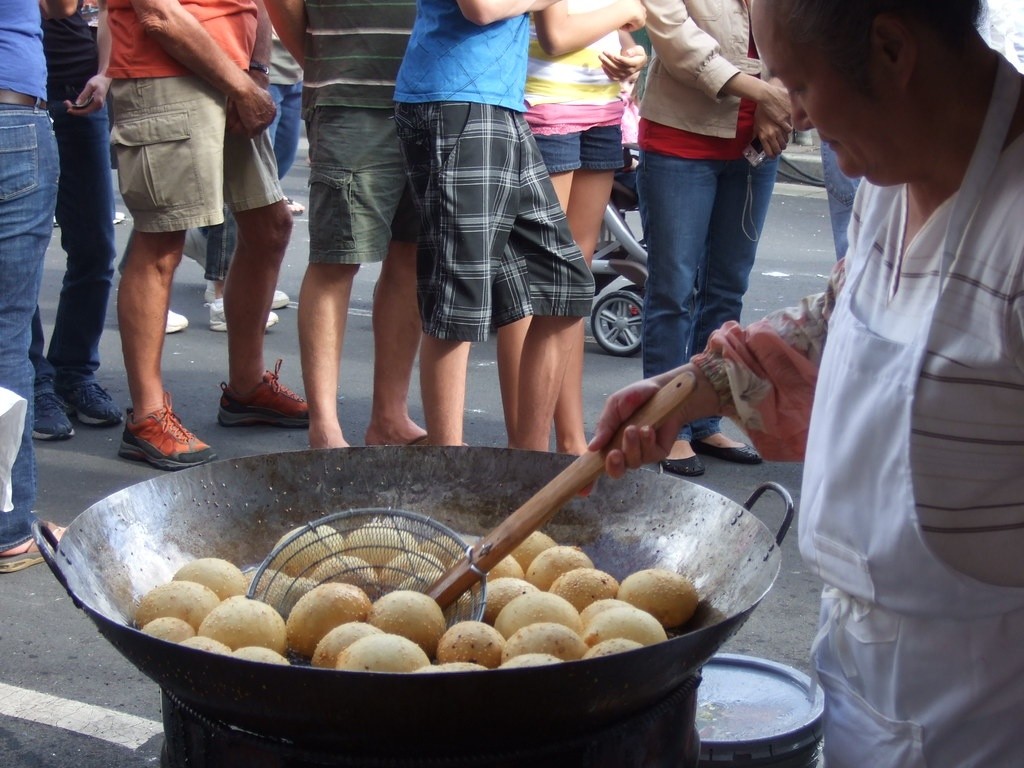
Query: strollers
587,142,651,356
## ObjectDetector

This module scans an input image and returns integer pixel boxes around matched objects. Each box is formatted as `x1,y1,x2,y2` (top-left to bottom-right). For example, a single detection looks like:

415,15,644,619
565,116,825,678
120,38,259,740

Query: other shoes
691,439,762,464
165,309,188,333
657,454,705,476
113,212,125,225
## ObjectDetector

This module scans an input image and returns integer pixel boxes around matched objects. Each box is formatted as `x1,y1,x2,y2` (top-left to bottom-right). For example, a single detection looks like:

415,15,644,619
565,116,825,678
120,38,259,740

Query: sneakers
210,303,279,331
218,359,310,428
118,391,216,471
204,280,289,309
33,393,75,440
54,380,123,425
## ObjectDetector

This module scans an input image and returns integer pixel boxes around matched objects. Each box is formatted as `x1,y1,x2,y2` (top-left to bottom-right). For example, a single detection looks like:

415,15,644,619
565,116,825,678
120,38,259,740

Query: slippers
0,522,66,572
284,198,304,215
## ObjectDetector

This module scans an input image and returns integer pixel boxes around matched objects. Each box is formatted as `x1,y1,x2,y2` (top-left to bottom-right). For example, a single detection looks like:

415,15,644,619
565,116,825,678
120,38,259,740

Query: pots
37,446,797,741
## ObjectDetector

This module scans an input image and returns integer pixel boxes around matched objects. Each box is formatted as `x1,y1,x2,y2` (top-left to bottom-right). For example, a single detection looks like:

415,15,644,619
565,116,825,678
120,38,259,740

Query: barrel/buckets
694,653,827,766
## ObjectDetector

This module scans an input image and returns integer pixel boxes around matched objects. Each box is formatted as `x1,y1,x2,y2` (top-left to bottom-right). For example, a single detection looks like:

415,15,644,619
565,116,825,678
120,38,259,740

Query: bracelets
249,61,270,75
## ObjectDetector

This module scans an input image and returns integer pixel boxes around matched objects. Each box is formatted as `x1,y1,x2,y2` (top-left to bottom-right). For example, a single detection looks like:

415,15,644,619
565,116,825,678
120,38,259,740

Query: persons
0,0,863,573
579,0,1024,768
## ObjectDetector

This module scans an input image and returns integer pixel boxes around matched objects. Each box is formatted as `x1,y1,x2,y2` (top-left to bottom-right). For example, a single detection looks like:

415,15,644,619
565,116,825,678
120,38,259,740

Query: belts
0,90,46,110
